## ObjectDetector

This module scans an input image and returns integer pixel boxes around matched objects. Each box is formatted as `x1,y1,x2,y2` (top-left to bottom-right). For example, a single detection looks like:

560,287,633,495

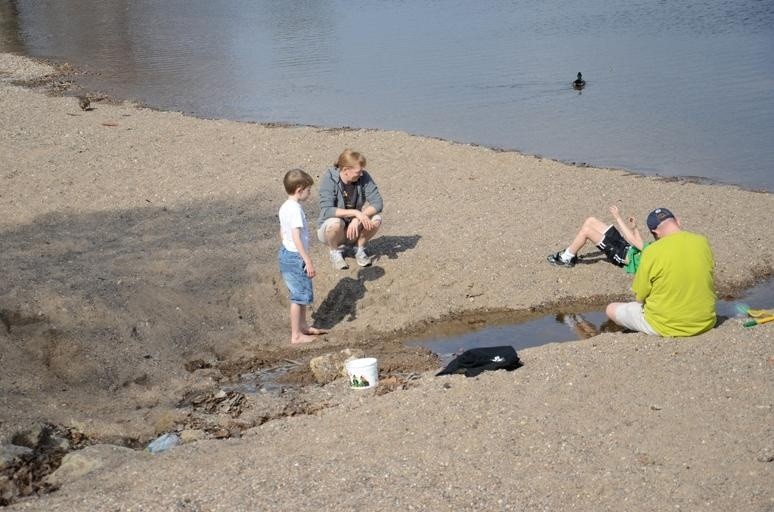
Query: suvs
348,357,380,391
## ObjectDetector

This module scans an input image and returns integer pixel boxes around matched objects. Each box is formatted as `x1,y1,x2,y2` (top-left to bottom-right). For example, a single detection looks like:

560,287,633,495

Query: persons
603,207,716,337
545,205,653,275
554,311,622,339
314,152,384,269
278,165,329,345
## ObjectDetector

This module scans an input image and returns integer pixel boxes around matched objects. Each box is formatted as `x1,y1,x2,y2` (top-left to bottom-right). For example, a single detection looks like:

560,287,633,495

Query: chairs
546,247,579,268
329,250,349,270
351,245,372,267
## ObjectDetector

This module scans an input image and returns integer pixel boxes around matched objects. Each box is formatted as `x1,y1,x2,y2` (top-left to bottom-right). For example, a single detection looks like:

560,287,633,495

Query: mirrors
80,98,90,111
573,72,585,89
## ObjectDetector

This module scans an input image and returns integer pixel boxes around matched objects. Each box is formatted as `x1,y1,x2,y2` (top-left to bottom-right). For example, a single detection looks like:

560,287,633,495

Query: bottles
646,207,674,233
